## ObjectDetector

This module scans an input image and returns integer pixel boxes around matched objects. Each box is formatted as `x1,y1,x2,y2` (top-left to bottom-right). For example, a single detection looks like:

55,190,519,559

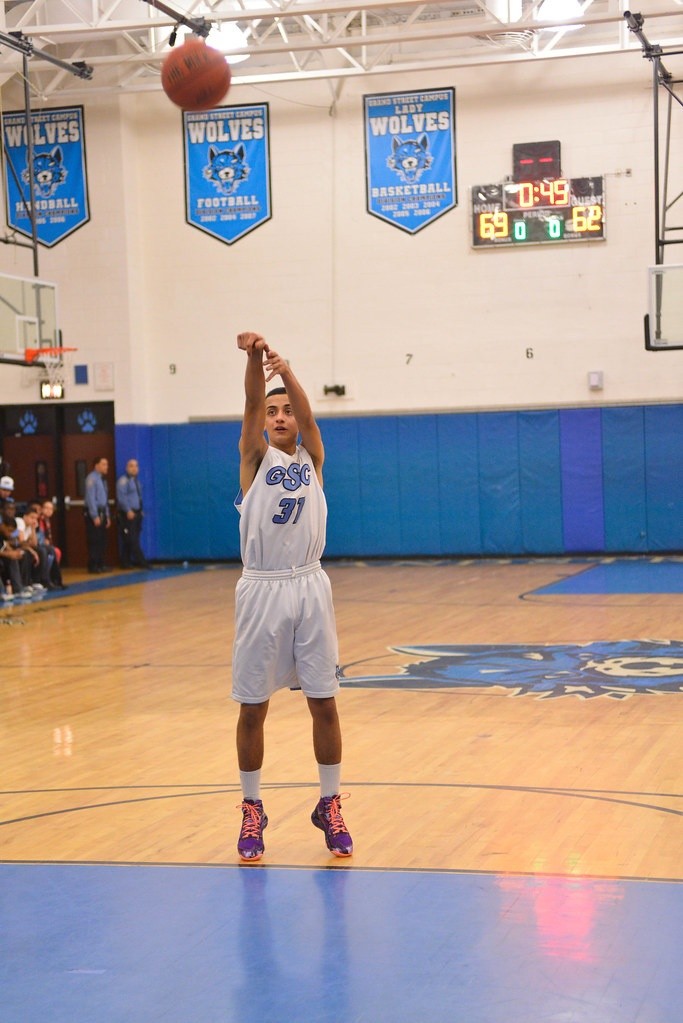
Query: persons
83,455,112,575
0,456,69,600
115,459,153,570
230,331,354,861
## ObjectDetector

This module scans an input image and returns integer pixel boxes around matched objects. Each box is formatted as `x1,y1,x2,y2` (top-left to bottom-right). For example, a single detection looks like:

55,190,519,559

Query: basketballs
160,42,231,112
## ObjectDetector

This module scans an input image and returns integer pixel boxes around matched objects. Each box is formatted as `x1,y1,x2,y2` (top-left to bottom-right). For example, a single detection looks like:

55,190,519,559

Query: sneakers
312,791,353,857
235,797,268,861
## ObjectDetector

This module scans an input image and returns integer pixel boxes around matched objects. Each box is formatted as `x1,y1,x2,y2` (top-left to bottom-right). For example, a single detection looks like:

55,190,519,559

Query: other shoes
88,565,112,572
0,579,64,602
133,560,150,568
120,563,135,569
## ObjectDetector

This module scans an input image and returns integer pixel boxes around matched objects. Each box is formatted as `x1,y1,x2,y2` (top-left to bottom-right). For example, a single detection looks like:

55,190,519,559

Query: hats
0,476,15,491
